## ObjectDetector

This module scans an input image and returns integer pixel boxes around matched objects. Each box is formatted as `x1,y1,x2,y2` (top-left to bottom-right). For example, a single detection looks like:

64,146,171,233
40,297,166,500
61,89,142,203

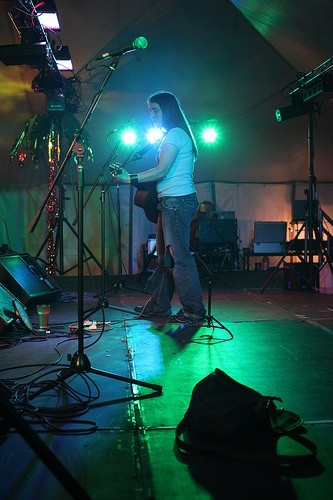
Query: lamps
56,45,74,71
275,93,319,122
35,0,61,32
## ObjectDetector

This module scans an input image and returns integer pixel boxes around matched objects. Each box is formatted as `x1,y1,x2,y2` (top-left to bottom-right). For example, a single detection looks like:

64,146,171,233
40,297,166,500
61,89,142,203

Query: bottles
263,256,269,271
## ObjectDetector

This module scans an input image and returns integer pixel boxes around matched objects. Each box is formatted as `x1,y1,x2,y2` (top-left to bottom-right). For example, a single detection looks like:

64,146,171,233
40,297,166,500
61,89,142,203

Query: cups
255,263,260,271
37,304,50,328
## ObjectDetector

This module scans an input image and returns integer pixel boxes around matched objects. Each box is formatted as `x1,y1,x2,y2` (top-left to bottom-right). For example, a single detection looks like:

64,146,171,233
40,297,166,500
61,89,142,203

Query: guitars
108,161,161,224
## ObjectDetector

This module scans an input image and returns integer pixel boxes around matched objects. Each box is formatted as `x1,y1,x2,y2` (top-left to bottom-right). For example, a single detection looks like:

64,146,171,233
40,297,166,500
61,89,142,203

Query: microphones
45,279,56,290
113,119,136,133
95,36,148,60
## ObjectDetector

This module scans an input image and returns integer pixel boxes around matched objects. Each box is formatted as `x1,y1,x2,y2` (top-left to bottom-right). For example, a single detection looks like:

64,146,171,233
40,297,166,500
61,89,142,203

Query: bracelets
130,174,139,186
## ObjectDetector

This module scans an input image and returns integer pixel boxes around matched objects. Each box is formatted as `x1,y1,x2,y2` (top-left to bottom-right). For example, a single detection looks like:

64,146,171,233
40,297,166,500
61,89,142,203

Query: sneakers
134,305,172,317
175,314,204,324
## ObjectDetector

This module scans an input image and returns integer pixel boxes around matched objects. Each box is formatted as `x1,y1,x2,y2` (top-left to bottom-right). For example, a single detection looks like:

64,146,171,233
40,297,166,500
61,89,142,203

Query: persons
112,93,207,324
191,201,224,271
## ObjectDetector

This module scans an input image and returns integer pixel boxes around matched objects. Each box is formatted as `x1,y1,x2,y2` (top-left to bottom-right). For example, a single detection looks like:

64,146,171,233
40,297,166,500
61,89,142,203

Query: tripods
262,118,333,291
21,58,161,405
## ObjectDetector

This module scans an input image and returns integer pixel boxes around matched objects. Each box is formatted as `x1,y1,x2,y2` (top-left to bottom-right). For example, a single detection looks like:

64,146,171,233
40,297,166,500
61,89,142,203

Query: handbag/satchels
174,368,318,469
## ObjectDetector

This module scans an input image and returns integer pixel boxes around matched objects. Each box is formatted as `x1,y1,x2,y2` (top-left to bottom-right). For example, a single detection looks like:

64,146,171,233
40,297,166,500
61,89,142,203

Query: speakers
0,255,62,333
